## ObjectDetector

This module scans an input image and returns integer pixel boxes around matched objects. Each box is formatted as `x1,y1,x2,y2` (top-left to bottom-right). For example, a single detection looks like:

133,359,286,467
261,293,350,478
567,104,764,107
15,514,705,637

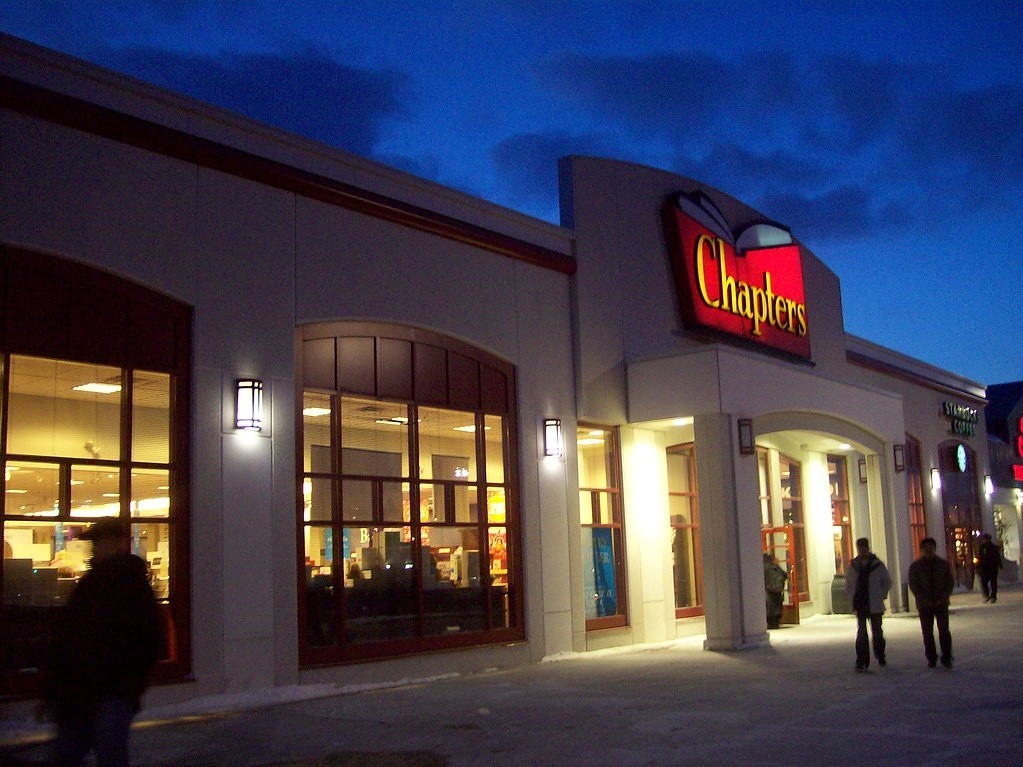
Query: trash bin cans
831,574,855,614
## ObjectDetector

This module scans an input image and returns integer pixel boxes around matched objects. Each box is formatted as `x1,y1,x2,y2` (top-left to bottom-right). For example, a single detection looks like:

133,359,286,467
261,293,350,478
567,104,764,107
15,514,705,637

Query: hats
78,518,128,540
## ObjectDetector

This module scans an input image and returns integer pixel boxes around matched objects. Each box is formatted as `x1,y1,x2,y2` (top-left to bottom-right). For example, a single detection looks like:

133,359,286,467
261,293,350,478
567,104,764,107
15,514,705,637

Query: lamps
984,474,994,495
858,459,868,485
236,377,263,431
893,443,905,472
544,417,563,458
931,467,942,492
737,417,757,455
374,419,404,427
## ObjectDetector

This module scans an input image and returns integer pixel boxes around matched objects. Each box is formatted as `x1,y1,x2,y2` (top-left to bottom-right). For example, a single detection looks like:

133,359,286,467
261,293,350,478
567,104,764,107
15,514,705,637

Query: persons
763,555,788,629
844,538,893,672
907,538,953,669
49,516,157,767
978,533,1004,603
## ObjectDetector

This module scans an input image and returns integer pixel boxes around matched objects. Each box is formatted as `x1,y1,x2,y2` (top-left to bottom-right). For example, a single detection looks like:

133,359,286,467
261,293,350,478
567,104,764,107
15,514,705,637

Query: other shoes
929,656,938,667
987,598,990,601
940,655,952,667
991,598,995,603
